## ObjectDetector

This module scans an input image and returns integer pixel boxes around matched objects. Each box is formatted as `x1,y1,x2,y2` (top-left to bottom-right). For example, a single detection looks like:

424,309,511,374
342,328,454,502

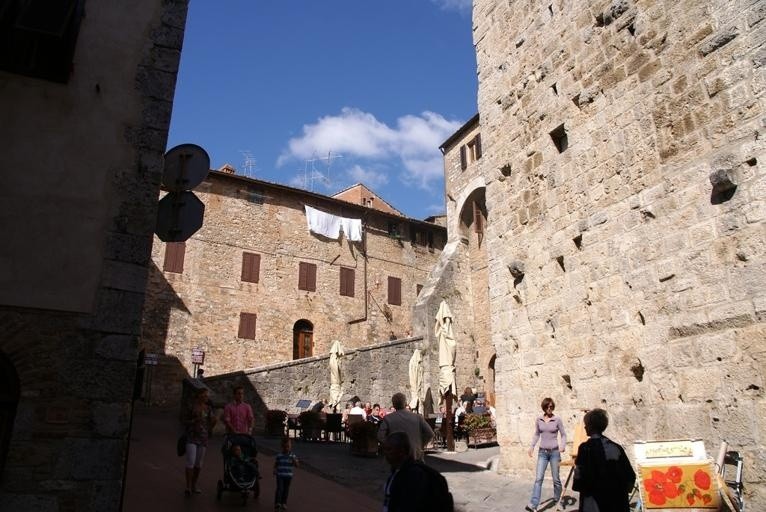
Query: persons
440,387,497,438
223,385,256,482
272,438,299,512
525,398,566,511
572,408,636,512
198,369,203,378
404,332,411,340
381,431,455,511
232,445,264,482
207,399,214,438
390,331,397,341
311,396,412,456
180,388,218,497
375,393,434,471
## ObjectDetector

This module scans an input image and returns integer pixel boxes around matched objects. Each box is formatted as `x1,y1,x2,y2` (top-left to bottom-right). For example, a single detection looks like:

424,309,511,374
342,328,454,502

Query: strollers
216,432,264,507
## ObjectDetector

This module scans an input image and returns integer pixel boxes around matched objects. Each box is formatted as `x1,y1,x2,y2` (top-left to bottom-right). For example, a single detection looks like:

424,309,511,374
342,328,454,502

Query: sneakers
275,503,288,511
238,474,264,482
555,502,564,511
184,487,201,497
525,504,537,512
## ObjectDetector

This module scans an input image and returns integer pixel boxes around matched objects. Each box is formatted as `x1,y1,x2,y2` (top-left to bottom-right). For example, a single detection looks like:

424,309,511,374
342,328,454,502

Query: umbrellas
434,300,458,396
328,340,344,440
409,348,425,416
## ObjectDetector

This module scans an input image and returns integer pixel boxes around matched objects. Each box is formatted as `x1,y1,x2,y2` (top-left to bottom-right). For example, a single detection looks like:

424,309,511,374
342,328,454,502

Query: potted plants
464,412,497,450
350,418,381,458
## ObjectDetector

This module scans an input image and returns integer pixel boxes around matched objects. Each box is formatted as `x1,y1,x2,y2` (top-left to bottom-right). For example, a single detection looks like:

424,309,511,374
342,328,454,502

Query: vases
264,422,286,440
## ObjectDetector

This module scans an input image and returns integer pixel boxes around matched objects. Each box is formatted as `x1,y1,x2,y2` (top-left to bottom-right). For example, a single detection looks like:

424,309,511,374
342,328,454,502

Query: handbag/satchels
177,433,186,456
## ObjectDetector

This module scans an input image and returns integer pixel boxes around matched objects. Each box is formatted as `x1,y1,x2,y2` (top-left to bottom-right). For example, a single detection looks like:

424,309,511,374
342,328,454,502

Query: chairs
424,415,469,454
287,413,363,448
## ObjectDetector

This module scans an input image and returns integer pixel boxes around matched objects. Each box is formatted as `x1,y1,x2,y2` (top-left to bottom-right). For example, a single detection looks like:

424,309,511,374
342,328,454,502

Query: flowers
263,409,288,423
296,410,322,424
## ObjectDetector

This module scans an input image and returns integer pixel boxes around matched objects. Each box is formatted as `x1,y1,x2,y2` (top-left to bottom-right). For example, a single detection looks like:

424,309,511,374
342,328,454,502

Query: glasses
544,406,554,410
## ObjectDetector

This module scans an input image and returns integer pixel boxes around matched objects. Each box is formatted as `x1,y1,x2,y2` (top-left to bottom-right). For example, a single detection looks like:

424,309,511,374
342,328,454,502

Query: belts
540,446,558,452
